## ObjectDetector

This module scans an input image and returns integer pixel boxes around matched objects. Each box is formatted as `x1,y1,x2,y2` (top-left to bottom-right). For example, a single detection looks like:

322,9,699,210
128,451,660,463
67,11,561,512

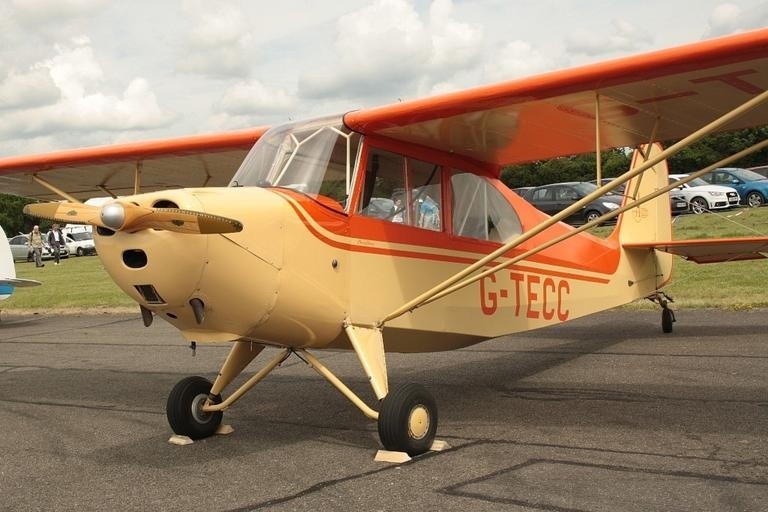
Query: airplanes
0,27,768,454
0,225,42,300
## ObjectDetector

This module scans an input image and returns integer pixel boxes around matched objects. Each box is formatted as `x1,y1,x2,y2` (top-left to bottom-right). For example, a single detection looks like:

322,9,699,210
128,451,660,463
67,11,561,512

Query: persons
50,223,66,267
419,195,440,231
28,224,47,268
390,199,404,223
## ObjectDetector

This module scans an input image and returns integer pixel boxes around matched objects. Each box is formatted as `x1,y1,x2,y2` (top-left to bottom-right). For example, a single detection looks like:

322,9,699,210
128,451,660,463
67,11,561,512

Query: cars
368,197,395,221
7,223,95,263
510,165,768,228
